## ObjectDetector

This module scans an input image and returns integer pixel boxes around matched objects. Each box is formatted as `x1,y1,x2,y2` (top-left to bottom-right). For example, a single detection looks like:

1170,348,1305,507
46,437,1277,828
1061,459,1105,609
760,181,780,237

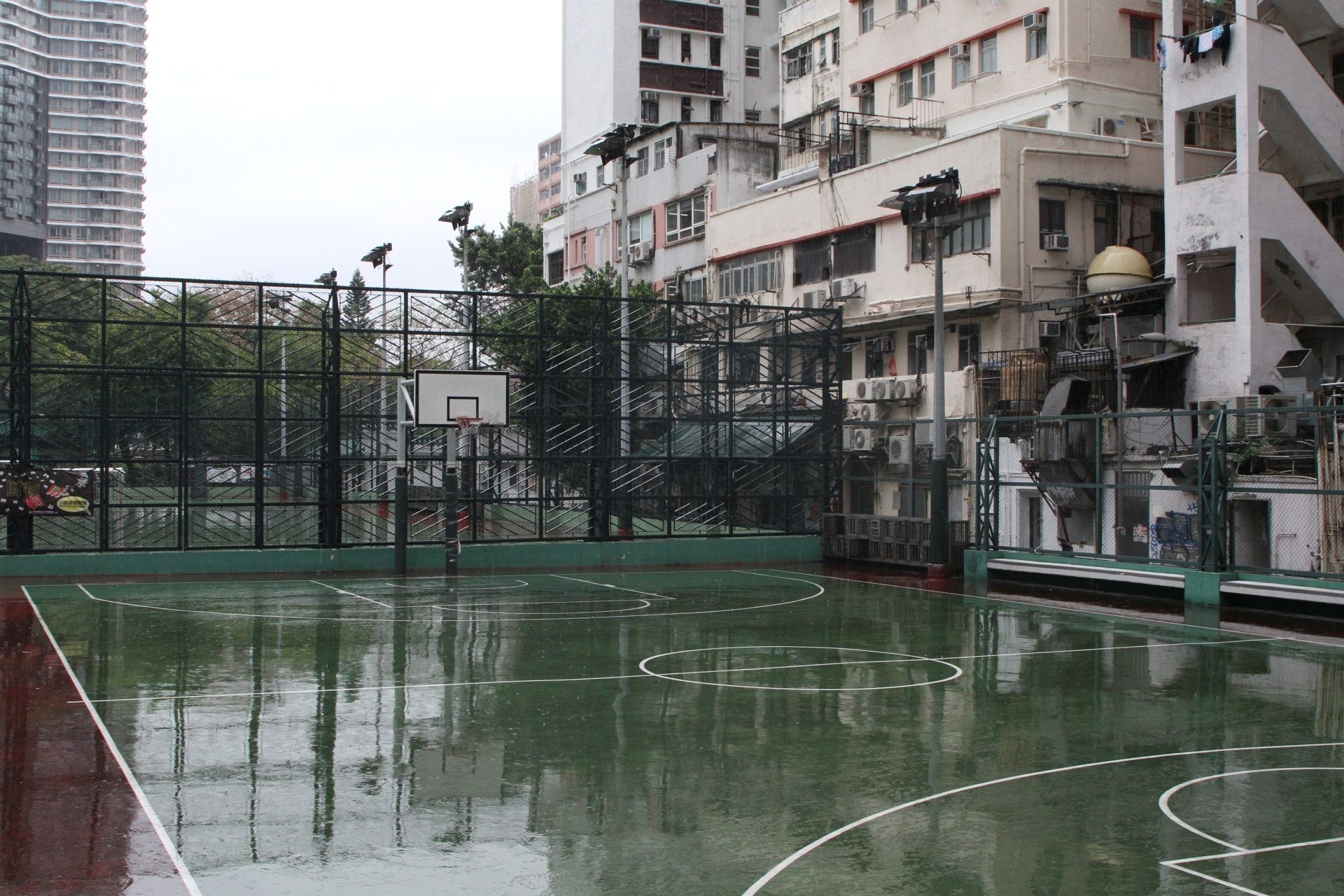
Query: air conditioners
1045,234,1069,252
832,278,859,298
628,254,634,264
635,244,651,261
1096,116,1125,139
876,380,895,399
803,290,827,310
759,391,773,405
887,435,913,464
861,403,882,422
1023,13,1046,28
1040,322,1061,337
574,174,583,183
850,44,971,97
895,380,917,399
1197,397,1247,438
876,335,935,354
648,28,663,38
855,379,875,400
1245,394,1298,436
644,92,657,100
854,429,881,449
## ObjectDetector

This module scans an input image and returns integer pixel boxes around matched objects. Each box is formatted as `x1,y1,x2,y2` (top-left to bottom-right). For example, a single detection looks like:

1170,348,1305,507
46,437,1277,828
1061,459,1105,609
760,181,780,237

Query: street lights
268,295,292,502
438,201,478,528
583,124,639,538
877,165,963,565
359,242,394,520
